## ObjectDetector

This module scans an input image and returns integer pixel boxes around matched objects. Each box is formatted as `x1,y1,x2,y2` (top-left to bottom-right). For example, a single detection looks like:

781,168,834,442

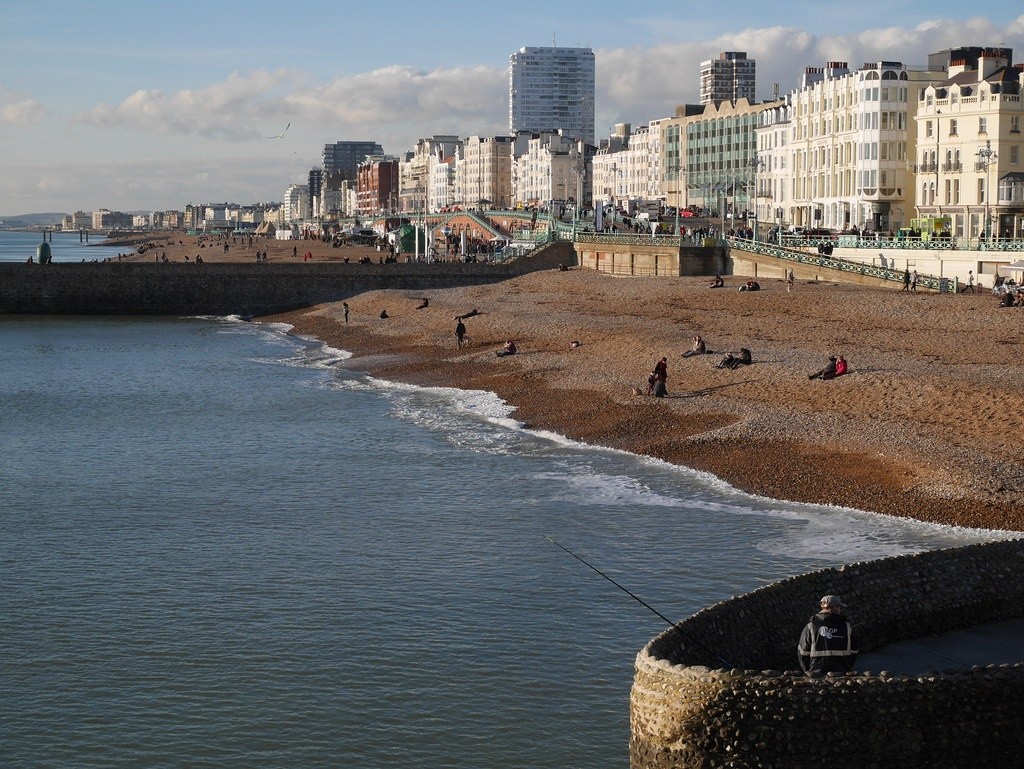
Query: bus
911,218,951,249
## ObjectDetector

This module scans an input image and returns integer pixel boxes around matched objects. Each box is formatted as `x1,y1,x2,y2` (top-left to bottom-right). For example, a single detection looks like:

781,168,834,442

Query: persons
496,340,517,356
807,355,846,379
998,279,1024,307
416,298,429,310
709,275,723,287
739,280,760,291
797,595,858,672
960,270,975,293
455,319,466,350
454,309,477,319
786,269,793,292
648,357,668,398
27,201,1024,263
379,310,389,319
716,348,752,370
902,269,917,291
681,336,706,357
343,302,349,324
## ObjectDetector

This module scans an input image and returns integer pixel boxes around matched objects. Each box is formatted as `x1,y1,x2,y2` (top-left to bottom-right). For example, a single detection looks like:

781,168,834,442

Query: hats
820,596,848,608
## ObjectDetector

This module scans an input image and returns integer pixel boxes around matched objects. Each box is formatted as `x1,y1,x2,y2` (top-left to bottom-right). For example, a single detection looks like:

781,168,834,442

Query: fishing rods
542,532,736,670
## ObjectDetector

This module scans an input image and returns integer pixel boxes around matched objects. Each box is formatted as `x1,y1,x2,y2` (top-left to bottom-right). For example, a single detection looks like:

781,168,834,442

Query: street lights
748,151,765,246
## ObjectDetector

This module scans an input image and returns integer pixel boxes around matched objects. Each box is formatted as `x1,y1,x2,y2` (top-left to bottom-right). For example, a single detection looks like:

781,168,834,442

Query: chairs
994,285,1019,297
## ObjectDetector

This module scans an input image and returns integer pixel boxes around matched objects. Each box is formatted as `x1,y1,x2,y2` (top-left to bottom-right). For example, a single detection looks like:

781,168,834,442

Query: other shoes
807,374,812,380
495,350,502,357
726,365,734,371
715,365,724,369
681,354,687,358
